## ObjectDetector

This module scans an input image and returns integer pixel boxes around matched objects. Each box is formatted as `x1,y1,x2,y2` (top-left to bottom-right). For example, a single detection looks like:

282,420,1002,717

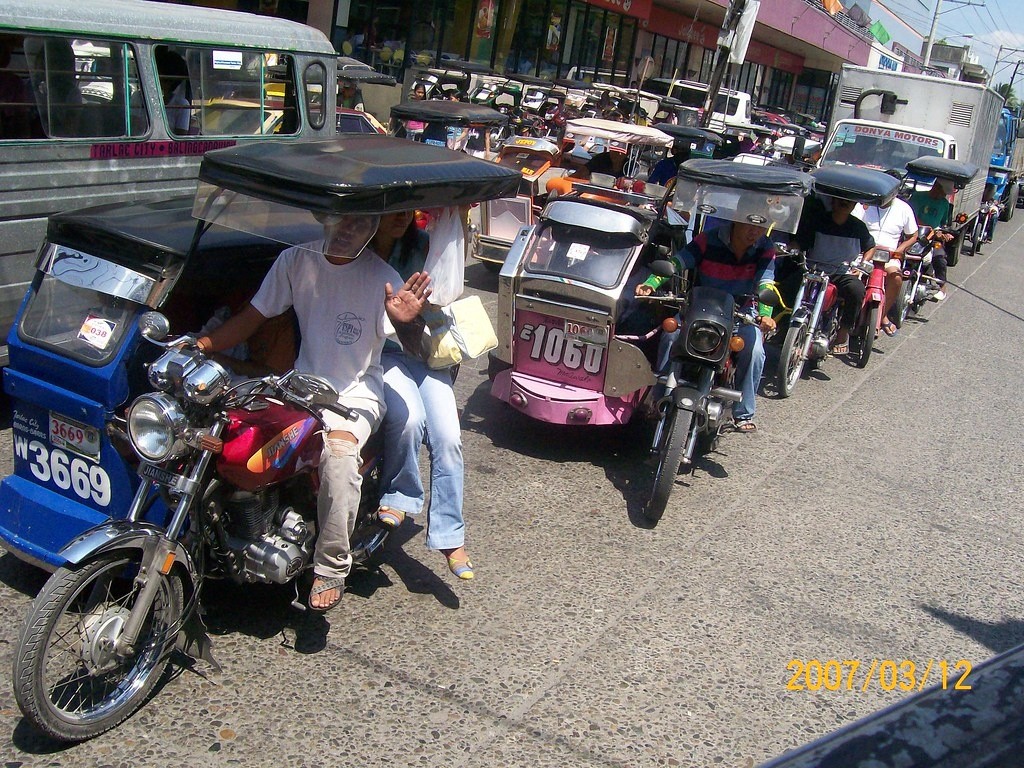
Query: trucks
814,62,1006,268
981,106,1024,222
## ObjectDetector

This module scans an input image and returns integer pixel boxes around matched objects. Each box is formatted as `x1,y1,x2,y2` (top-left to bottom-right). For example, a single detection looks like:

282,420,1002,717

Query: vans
0,1,340,350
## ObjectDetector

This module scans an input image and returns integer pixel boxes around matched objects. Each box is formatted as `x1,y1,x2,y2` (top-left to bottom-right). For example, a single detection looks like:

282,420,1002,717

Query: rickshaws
0,131,527,743
480,155,816,524
251,53,1004,399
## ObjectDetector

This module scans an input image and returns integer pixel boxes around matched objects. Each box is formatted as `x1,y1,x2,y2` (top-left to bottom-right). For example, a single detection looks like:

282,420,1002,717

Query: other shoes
439,549,474,580
933,291,946,300
378,505,405,528
987,237,993,244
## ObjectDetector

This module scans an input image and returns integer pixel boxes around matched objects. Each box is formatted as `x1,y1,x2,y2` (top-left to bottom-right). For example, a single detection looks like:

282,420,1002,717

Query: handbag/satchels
422,205,465,306
420,295,499,370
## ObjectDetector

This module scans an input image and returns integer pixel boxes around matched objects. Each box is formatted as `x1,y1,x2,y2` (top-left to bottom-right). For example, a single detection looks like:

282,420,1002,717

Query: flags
820,0,844,17
868,20,891,45
847,2,872,27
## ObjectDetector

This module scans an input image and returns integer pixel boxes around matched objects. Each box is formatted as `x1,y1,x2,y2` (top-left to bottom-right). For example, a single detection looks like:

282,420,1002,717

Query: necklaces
877,207,891,230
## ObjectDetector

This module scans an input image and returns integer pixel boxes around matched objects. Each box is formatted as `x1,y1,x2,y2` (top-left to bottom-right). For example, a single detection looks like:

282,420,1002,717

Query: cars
187,99,388,136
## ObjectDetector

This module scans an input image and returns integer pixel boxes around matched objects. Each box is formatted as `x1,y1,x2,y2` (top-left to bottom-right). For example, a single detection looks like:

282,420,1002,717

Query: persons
785,195,875,355
176,208,434,612
850,170,918,335
908,178,955,302
608,93,640,123
338,76,491,161
292,208,474,582
636,190,776,432
646,140,692,195
567,144,635,187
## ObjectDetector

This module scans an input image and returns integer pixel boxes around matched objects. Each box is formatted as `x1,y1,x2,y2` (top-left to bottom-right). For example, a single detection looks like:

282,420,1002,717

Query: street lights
921,32,975,77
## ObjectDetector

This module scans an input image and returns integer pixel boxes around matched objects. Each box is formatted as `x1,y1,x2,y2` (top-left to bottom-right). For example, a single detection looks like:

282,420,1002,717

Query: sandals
308,573,344,611
831,333,850,355
880,321,898,338
735,417,757,434
769,328,788,347
646,400,665,422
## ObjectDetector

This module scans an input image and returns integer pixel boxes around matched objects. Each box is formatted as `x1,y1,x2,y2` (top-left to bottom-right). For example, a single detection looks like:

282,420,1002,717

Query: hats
937,177,955,195
